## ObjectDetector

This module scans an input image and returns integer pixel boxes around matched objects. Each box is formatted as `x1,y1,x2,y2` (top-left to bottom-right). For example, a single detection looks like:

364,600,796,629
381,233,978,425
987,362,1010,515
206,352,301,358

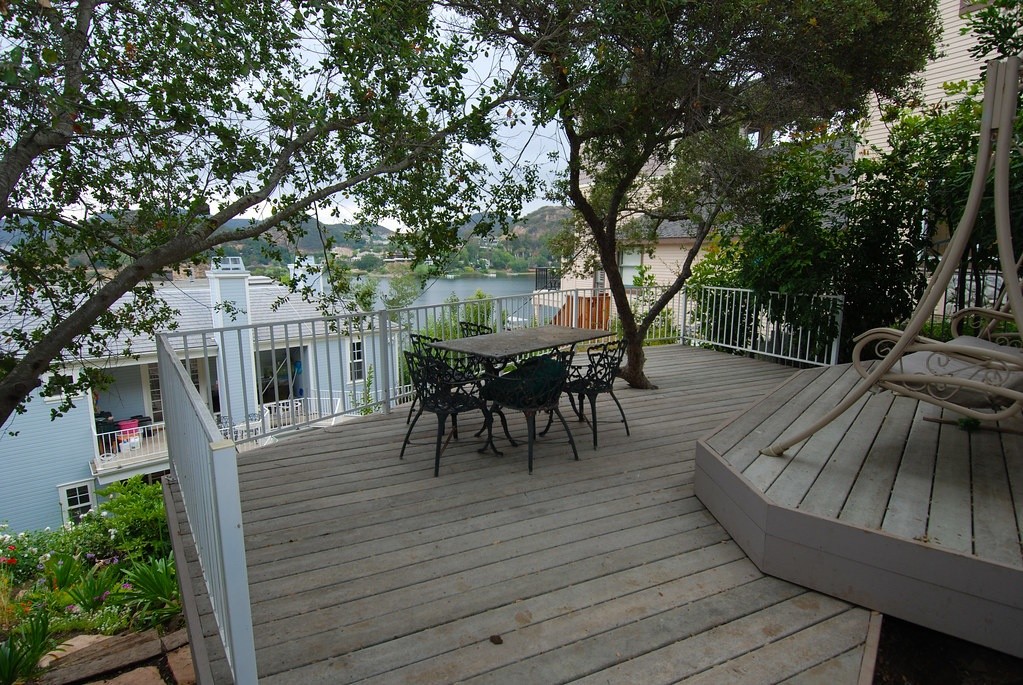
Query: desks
426,325,618,448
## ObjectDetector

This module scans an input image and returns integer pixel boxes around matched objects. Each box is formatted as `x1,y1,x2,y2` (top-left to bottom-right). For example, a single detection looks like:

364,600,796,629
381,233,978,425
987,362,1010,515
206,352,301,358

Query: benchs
756,254,1022,456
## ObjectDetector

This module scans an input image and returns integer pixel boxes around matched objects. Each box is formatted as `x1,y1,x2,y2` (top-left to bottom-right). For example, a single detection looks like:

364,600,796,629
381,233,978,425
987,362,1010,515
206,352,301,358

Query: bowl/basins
118,419,138,433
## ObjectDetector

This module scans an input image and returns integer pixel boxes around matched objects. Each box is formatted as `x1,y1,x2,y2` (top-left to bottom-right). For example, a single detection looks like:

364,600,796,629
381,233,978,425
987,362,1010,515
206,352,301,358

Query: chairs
399,321,630,479
216,413,261,453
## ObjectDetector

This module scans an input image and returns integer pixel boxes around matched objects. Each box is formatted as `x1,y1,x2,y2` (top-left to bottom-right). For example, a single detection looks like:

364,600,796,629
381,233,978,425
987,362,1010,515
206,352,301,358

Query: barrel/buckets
120,439,130,453
129,436,140,450
